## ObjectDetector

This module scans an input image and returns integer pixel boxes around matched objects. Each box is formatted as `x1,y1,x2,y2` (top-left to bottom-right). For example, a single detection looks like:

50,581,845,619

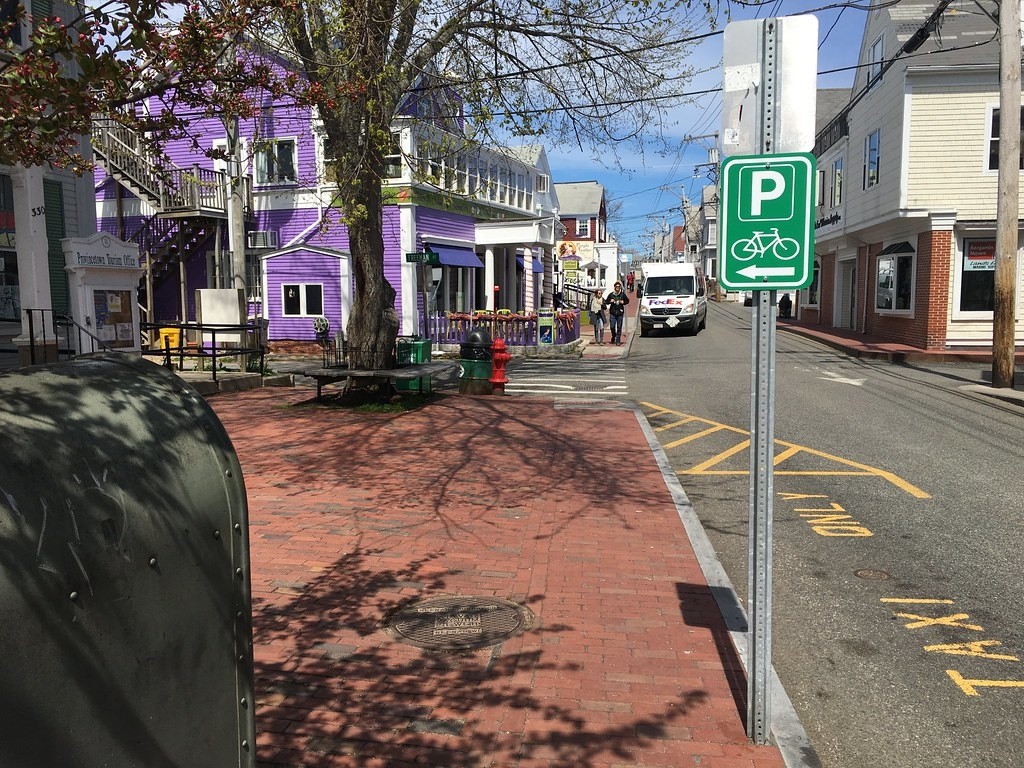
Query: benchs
278,361,348,398
396,363,454,396
305,368,423,398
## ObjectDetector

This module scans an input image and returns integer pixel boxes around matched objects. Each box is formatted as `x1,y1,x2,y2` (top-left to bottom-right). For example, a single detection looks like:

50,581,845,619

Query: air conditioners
248,231,278,248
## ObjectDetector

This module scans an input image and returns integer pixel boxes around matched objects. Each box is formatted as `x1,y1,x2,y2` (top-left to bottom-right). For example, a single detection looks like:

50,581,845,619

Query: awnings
429,246,484,268
518,258,544,273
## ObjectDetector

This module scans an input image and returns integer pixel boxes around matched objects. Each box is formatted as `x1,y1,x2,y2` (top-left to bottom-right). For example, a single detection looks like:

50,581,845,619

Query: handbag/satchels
590,314,597,325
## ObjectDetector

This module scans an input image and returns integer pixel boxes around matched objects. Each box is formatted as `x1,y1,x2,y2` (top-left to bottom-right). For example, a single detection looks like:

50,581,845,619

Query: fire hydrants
488,338,513,395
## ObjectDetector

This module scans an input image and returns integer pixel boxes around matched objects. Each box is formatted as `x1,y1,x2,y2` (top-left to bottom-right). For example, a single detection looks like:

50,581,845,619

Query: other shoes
632,290,633,292
630,292,631,293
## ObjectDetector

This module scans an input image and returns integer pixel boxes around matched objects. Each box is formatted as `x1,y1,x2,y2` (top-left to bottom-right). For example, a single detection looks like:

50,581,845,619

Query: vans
637,263,707,334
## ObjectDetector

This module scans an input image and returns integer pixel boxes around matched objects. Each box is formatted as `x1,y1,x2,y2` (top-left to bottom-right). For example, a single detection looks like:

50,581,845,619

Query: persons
628,271,635,293
590,290,610,346
705,275,711,287
606,282,629,346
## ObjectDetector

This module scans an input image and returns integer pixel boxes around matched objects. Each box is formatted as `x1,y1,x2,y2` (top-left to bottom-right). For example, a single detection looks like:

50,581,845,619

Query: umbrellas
581,261,608,279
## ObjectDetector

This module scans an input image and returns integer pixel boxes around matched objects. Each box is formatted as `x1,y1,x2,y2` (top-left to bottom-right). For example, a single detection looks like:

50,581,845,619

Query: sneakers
600,342,604,346
611,337,615,343
616,339,620,345
595,342,598,346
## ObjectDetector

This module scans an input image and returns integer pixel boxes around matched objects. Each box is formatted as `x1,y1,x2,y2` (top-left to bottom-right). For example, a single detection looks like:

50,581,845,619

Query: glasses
599,293,603,295
616,286,620,288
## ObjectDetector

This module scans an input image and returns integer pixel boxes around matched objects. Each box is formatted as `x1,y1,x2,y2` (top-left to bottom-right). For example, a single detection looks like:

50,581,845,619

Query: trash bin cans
459,328,495,395
1,348,257,768
158,328,181,355
396,338,432,390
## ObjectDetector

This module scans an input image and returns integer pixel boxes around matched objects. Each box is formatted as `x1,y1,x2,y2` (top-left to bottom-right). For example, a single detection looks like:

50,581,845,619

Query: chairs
444,309,528,342
